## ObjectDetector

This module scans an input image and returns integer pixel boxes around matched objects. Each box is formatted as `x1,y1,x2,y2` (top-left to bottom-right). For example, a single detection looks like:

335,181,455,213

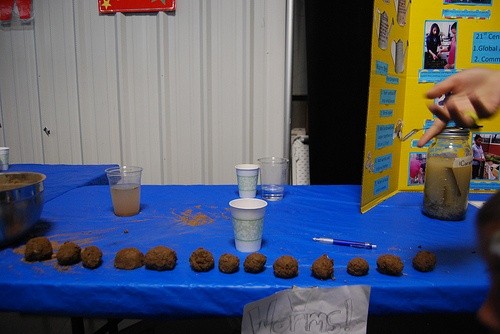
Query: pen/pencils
313,235,377,251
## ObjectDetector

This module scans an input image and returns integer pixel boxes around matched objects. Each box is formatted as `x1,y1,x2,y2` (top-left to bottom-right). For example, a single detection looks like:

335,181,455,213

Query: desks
0,184,495,334
0,163,121,205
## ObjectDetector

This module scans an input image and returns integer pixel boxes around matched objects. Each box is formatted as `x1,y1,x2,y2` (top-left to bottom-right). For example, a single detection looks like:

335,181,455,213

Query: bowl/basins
0,172,47,248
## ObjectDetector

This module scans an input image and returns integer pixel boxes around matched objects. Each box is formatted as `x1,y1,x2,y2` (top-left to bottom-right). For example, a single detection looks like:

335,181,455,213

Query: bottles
421,127,474,222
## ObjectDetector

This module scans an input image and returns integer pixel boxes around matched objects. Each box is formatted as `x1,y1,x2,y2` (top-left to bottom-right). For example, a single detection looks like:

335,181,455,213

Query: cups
234,164,261,198
104,166,144,217
257,156,290,202
0,146,10,171
228,198,269,253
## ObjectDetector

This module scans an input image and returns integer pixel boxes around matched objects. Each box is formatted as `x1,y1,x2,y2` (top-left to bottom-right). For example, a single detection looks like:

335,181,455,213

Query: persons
417,66,500,148
472,135,485,179
426,22,455,69
447,192,500,334
410,154,426,184
